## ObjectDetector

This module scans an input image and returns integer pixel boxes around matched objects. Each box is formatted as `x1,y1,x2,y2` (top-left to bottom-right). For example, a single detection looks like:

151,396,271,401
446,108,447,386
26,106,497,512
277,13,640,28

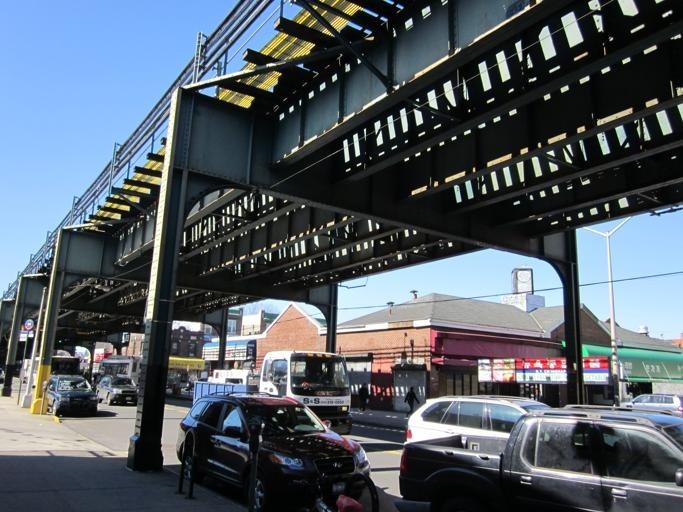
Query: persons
404,386,420,416
359,383,369,412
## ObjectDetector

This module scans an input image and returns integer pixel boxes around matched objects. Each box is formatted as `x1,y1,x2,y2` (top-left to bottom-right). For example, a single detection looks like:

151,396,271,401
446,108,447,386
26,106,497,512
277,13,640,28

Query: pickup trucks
398,411,683,511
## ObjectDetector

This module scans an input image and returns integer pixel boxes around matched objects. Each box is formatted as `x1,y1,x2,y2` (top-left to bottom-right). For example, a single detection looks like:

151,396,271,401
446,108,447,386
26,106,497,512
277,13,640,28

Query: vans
621,392,680,411
402,393,551,441
177,393,371,511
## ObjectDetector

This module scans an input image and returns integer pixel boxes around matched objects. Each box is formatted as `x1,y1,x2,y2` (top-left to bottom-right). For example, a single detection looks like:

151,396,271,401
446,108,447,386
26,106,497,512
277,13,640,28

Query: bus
30,357,80,387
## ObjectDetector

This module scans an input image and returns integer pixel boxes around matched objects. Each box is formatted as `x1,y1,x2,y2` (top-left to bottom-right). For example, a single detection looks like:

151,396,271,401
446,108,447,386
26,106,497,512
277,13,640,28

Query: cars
46,374,98,416
96,374,138,406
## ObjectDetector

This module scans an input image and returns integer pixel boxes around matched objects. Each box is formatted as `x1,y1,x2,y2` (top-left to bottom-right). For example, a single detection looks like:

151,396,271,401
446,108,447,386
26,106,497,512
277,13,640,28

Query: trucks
194,350,353,434
99,355,182,395
